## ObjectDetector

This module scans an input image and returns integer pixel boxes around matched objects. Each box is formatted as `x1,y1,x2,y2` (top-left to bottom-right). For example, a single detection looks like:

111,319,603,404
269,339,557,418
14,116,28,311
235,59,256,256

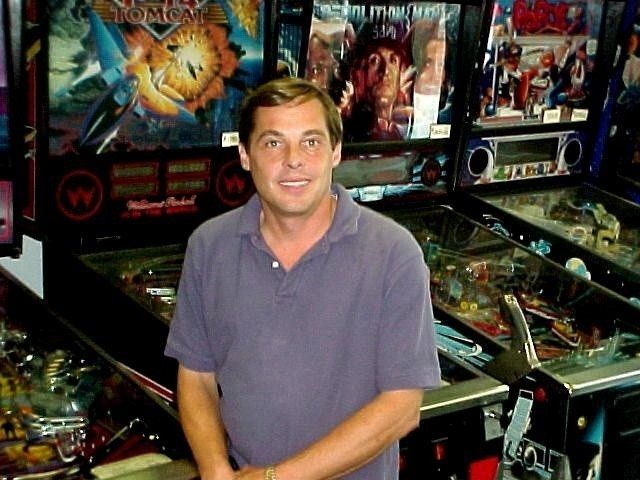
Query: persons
304,17,354,98
334,16,412,147
162,77,444,480
402,20,446,97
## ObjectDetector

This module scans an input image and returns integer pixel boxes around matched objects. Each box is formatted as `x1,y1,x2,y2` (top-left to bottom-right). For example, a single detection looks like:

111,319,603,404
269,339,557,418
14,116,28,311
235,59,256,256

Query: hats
352,14,408,57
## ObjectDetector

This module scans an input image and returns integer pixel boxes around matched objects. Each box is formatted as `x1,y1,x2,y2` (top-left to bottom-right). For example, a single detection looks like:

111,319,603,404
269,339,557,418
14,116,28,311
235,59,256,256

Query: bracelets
264,464,276,480
